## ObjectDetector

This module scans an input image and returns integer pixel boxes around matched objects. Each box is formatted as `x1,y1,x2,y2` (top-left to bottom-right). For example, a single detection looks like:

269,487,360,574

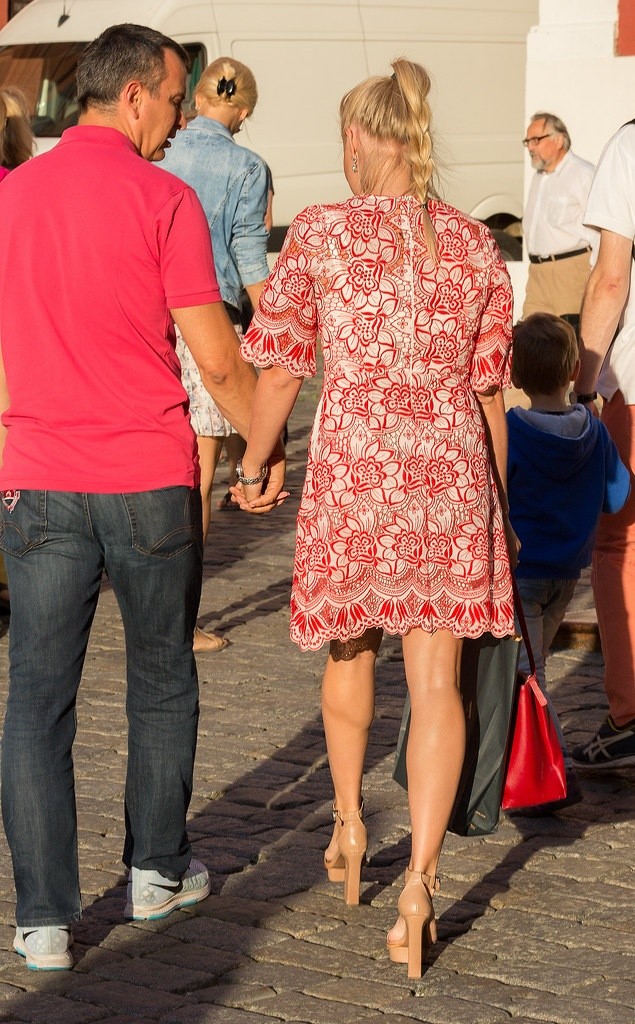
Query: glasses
522,134,551,147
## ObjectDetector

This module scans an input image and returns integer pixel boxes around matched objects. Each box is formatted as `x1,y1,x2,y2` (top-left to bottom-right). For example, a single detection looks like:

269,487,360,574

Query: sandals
191,625,228,653
216,492,240,510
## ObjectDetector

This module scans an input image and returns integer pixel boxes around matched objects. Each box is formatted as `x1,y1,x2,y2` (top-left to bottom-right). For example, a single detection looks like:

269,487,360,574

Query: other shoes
0,582,11,617
507,773,582,818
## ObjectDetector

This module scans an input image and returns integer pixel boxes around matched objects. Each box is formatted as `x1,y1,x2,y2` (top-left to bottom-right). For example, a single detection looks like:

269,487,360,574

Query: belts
529,246,591,264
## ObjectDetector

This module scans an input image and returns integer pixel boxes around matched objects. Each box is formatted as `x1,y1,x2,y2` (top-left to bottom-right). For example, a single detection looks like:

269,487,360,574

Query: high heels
386,866,438,979
324,795,368,906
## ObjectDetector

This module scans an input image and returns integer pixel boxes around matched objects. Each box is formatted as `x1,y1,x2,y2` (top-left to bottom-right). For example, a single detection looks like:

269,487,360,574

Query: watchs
569,391,597,405
235,459,267,484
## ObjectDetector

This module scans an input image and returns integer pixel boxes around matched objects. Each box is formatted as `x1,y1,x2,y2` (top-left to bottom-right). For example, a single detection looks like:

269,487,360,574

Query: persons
571,117,635,770
145,55,275,654
502,114,597,414
0,24,287,971
236,56,523,979
502,312,630,811
0,85,35,182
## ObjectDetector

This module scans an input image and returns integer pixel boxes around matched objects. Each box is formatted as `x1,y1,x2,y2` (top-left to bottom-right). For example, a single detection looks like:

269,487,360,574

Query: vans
0,0,542,278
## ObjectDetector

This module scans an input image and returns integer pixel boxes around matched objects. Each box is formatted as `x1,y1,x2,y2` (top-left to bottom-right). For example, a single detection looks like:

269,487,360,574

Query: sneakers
13,923,75,971
124,858,211,921
570,714,635,768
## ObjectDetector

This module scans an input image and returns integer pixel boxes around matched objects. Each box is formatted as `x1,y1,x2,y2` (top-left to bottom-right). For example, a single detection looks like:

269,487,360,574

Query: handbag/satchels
393,632,523,836
500,669,567,810
